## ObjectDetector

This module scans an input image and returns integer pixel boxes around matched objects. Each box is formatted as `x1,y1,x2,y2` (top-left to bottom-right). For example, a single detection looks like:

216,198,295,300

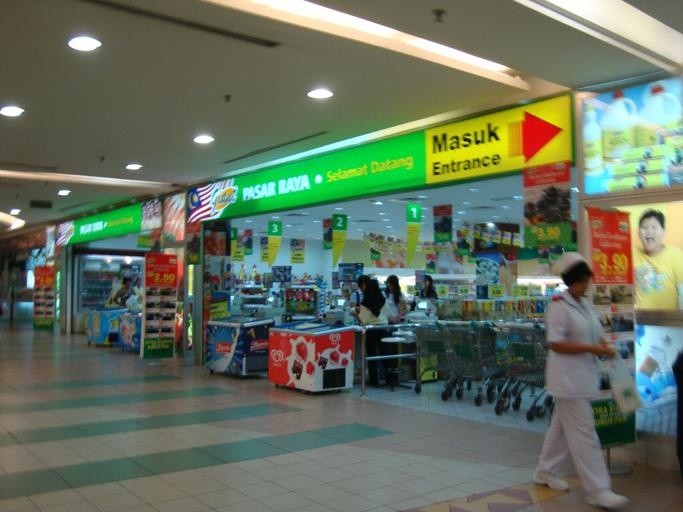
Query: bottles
250,264,257,282
239,264,247,280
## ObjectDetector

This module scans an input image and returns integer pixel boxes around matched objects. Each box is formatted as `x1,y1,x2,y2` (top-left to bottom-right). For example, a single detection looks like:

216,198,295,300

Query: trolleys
405,311,552,423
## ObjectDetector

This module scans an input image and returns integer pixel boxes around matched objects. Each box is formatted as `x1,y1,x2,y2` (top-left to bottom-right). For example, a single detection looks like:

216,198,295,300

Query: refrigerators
267,320,355,393
85,306,142,354
206,315,275,377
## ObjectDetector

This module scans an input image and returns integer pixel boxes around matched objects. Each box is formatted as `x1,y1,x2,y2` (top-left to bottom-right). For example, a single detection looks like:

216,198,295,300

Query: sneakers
534,471,570,491
586,489,628,508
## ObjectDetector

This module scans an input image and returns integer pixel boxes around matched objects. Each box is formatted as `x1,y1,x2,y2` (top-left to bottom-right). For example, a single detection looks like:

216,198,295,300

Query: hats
554,250,583,273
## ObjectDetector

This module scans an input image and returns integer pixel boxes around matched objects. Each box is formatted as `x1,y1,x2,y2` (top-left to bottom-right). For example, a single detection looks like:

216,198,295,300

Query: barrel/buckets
600,90,641,171
636,85,682,146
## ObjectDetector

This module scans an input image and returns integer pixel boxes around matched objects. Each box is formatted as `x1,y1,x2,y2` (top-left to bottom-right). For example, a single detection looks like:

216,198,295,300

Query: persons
527,258,634,508
383,274,409,365
628,207,682,313
130,276,144,297
113,276,135,306
410,272,439,315
349,279,400,389
349,274,369,325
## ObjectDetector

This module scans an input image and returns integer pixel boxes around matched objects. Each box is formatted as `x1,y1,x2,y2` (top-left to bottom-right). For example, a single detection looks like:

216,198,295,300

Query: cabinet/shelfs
238,284,275,311
